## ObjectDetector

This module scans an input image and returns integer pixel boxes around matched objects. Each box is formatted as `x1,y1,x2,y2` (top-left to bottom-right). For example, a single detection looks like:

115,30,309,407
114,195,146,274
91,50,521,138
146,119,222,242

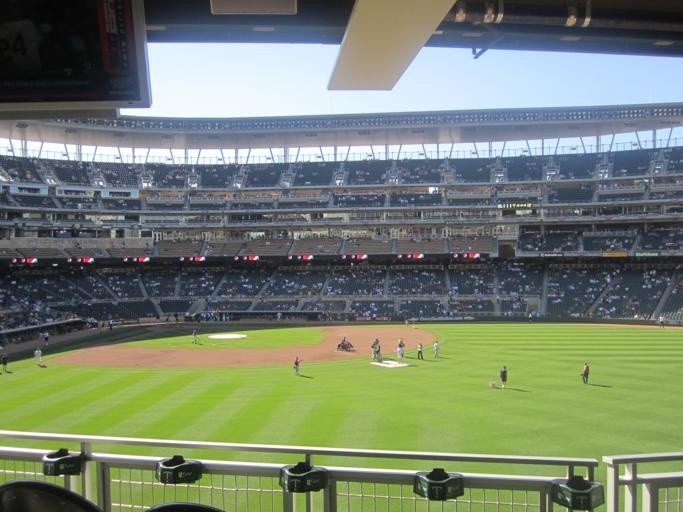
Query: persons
32,347,41,363
393,344,403,365
583,362,589,383
0,351,7,372
434,340,438,357
341,337,347,346
417,343,423,360
293,355,301,373
192,328,198,344
398,338,405,350
498,366,507,386
371,338,381,360
43,329,48,347
0,137,683,338
